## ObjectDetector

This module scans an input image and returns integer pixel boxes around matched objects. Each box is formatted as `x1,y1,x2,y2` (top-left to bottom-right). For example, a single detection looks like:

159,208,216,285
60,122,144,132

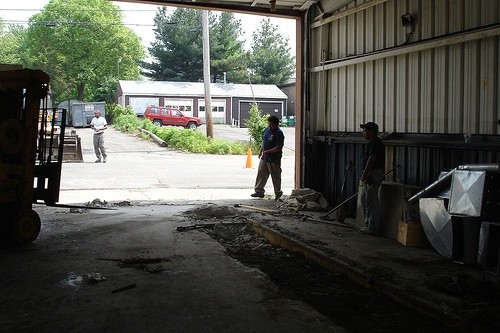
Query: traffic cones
242,148,255,169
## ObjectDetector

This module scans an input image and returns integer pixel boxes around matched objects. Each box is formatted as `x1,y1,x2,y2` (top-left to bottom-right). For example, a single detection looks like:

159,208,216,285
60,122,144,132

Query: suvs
142,105,202,129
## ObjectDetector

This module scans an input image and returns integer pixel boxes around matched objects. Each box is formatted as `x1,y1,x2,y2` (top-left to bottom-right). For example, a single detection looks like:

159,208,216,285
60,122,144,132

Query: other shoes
95,160,101,163
275,192,282,202
251,191,264,197
103,158,106,163
359,226,378,235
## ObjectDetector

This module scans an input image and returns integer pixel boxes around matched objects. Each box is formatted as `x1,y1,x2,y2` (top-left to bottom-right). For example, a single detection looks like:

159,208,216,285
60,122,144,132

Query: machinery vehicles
0,63,67,244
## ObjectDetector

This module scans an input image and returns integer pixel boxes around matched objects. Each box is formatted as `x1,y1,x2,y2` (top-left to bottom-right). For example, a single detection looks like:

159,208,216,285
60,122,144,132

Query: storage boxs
398,221,427,248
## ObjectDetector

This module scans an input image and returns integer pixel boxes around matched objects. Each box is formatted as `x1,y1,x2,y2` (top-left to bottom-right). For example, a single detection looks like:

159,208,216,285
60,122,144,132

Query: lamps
402,13,414,26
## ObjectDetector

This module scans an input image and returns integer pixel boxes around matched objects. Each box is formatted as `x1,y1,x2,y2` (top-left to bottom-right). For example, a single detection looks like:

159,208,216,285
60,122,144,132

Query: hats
360,122,378,132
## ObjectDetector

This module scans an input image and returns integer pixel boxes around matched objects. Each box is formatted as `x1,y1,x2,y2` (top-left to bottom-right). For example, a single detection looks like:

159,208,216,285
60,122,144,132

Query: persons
251,116,284,198
90,111,108,162
359,122,385,235
